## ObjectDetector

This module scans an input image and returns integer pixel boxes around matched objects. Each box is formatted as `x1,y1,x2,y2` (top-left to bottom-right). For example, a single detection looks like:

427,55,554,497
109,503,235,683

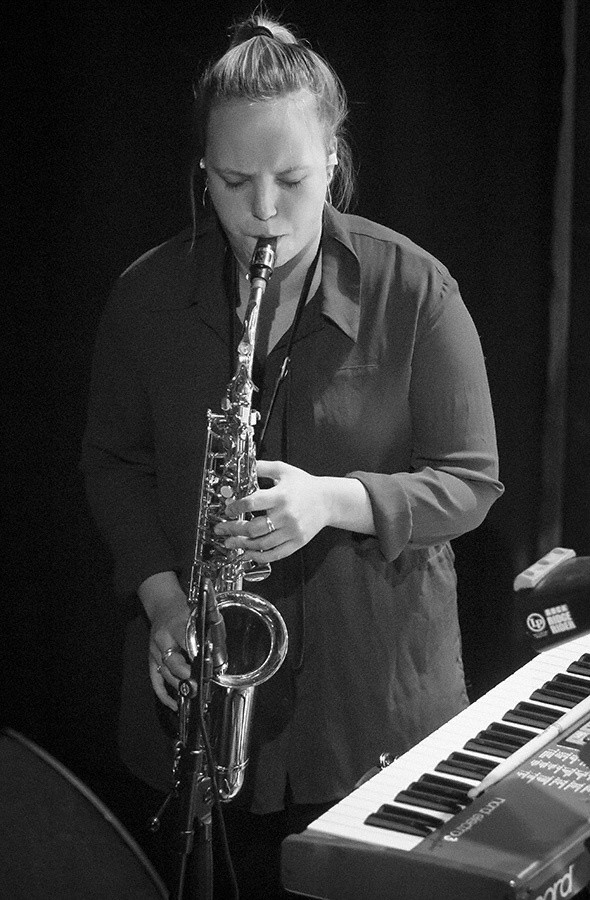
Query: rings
263,515,275,533
160,644,175,663
156,663,166,672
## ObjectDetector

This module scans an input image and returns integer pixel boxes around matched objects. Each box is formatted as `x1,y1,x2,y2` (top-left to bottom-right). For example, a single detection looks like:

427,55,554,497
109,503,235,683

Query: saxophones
173,235,290,802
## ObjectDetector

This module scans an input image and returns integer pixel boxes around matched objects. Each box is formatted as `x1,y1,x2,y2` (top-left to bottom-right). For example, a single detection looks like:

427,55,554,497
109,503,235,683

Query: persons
80,17,507,900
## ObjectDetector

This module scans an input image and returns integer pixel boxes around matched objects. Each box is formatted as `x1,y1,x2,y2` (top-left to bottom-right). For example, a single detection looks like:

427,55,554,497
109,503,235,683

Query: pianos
279,624,590,900
